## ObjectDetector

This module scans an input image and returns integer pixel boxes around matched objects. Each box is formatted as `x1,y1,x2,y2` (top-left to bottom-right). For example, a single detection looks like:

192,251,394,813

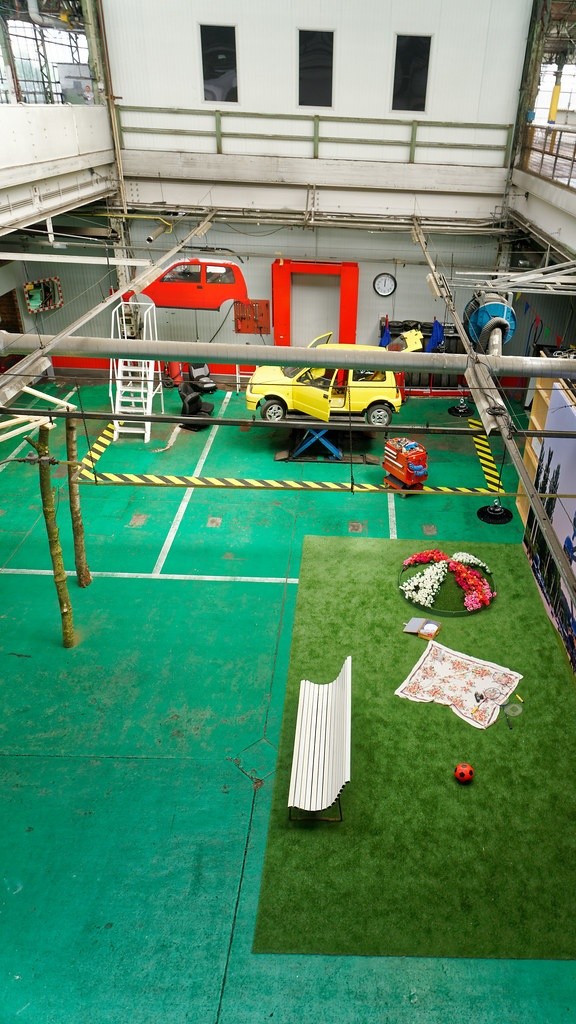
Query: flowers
400,549,498,611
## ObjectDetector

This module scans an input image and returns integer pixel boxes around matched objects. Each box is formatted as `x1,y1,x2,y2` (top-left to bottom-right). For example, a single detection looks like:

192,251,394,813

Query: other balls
453,763,474,782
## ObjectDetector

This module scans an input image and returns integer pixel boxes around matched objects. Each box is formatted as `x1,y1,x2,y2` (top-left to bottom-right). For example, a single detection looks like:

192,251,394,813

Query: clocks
374,273,398,296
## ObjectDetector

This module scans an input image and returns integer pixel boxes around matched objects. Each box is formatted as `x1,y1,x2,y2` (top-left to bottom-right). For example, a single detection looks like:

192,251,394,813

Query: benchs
287,656,352,823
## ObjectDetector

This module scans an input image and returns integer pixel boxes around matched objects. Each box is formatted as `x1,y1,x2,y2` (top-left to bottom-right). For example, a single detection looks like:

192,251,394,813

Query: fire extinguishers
168,360,182,385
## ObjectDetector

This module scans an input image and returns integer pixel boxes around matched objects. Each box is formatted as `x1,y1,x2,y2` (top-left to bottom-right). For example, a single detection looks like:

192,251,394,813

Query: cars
123,258,251,312
246,329,423,426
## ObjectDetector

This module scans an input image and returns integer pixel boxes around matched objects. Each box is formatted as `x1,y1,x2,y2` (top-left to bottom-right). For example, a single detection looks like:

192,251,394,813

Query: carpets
253,533,576,962
